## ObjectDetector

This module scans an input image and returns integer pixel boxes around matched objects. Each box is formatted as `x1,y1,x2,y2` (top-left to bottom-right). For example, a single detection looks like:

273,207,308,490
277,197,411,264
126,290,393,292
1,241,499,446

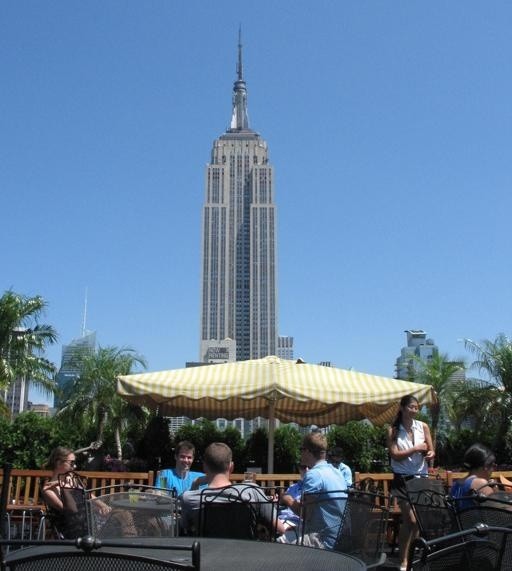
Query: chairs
1,473,512,571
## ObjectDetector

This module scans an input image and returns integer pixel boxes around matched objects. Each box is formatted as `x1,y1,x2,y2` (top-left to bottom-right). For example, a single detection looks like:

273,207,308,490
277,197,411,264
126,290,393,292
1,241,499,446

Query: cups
244,471,253,485
128,489,141,507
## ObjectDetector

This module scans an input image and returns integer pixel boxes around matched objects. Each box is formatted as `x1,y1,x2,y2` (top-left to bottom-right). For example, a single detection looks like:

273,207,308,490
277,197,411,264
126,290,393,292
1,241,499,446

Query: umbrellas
111,355,438,497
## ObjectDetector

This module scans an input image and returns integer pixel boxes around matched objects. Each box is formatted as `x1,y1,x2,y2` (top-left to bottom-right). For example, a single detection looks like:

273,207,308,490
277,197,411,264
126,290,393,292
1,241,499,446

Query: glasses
62,460,75,467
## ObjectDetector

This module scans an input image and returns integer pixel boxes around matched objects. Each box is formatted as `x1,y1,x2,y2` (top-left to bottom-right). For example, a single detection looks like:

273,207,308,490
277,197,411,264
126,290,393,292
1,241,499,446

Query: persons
448,444,494,516
179,443,285,544
43,447,138,540
149,442,208,537
277,433,353,551
385,396,436,571
